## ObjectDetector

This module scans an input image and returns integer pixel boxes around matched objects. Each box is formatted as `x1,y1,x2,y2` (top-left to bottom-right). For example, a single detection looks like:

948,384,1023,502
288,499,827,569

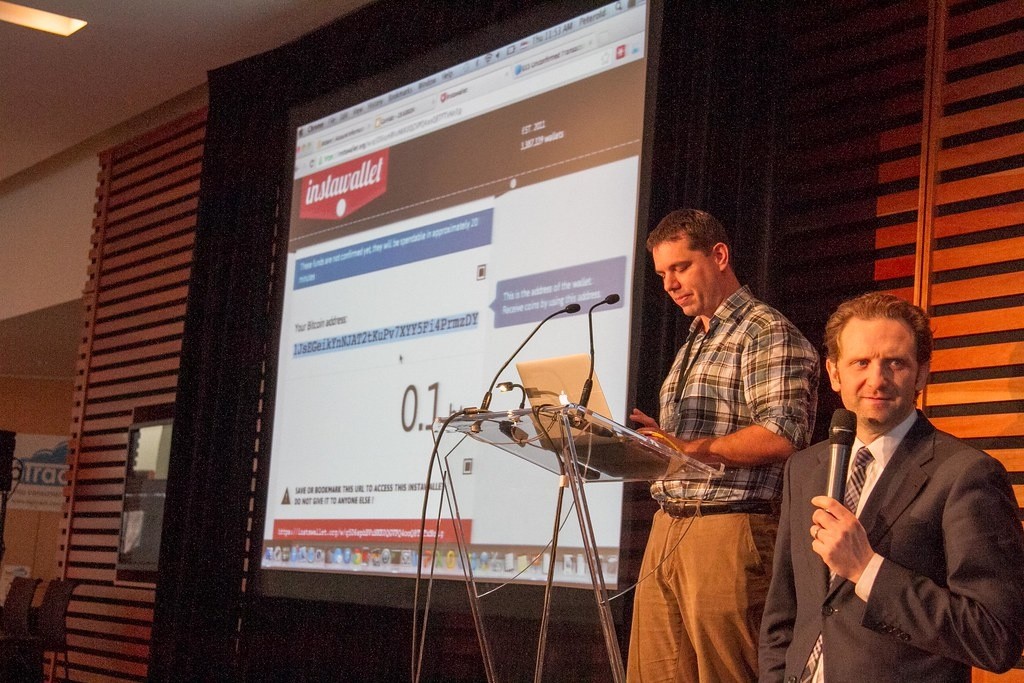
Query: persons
592,210,822,683
757,291,1023,683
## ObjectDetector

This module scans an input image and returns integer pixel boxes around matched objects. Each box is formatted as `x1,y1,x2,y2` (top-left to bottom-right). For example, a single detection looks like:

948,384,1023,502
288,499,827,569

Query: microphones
471,304,581,433
576,294,620,419
826,409,857,507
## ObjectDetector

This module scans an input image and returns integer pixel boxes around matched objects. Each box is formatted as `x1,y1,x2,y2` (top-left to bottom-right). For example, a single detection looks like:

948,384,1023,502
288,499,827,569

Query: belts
662,500,774,519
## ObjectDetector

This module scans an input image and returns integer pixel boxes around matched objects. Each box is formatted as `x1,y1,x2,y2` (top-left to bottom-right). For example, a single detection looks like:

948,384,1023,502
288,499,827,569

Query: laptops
514,355,676,477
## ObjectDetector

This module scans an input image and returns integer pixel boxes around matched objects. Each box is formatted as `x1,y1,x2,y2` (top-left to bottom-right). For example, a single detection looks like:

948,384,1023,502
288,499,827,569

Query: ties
796,445,876,681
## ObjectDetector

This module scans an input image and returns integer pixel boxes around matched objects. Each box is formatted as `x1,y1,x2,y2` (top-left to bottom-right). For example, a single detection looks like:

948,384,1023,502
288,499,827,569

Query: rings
815,526,822,539
